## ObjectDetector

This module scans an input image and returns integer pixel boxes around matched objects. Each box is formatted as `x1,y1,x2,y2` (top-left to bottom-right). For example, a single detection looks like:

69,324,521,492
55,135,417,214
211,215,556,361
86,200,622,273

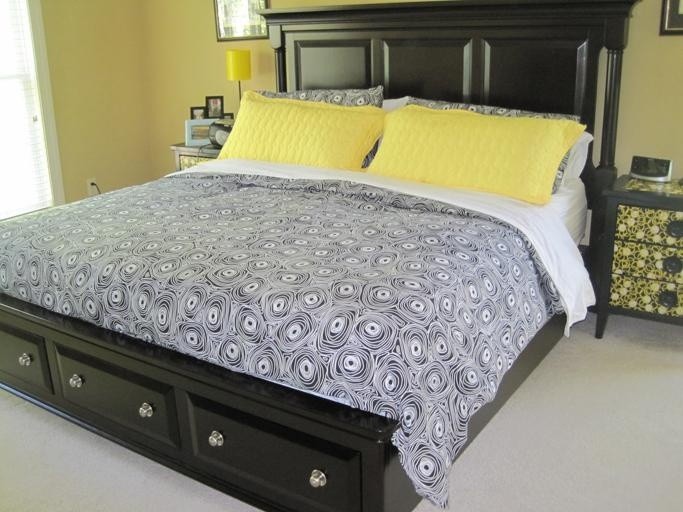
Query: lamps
225,47,251,108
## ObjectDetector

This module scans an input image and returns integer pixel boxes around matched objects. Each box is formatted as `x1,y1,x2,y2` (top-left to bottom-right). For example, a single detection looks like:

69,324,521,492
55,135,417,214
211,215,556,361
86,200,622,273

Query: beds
1,3,638,512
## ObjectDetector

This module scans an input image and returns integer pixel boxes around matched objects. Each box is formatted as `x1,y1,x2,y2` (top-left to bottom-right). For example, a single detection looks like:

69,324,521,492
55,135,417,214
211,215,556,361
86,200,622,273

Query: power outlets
84,176,98,195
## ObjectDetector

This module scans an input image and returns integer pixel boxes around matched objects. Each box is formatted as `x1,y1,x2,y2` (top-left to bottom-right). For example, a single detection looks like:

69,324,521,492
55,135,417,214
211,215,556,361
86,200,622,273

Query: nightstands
592,168,681,342
170,140,221,175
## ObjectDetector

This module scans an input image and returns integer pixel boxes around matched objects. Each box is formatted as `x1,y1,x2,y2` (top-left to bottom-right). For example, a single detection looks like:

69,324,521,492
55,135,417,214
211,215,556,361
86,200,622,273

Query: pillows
366,103,577,208
251,81,383,167
403,92,580,199
214,89,385,174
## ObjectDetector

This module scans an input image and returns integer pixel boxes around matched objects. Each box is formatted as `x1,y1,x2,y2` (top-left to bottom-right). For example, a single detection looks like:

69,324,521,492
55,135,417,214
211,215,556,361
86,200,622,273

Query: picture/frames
184,92,230,148
214,0,269,42
659,1,683,36
